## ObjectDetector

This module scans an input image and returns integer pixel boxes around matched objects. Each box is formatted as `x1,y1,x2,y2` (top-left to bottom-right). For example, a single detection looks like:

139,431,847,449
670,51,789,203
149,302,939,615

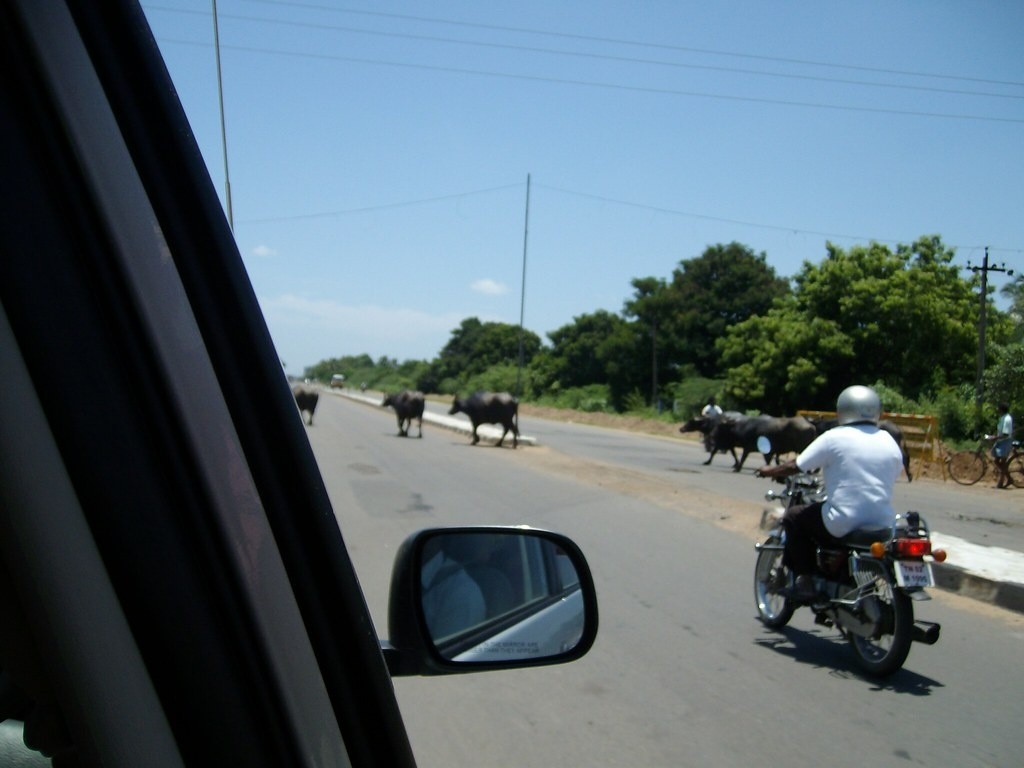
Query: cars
0,0,601,768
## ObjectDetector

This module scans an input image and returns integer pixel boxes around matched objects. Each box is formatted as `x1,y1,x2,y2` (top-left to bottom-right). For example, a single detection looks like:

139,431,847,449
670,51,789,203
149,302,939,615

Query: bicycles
948,433,1024,490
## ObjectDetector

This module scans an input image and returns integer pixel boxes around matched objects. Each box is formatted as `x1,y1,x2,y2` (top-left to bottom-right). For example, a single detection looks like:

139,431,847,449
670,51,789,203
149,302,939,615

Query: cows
292,385,321,426
449,389,521,448
678,412,913,483
379,389,425,438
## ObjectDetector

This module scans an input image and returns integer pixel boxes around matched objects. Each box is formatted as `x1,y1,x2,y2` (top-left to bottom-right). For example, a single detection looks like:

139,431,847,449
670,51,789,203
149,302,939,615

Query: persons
702,396,723,453
421,536,486,643
754,385,903,602
989,403,1014,489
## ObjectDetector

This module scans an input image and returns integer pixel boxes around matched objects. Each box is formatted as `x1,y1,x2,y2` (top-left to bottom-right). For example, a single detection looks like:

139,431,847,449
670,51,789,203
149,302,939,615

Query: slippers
776,575,813,597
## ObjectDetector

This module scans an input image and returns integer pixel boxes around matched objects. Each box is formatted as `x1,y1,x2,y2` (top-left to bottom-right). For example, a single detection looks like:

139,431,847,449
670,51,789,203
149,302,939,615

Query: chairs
450,544,519,613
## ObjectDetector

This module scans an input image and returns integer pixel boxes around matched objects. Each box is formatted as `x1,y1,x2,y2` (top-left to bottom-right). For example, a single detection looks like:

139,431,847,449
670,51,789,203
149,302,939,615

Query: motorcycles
751,435,947,677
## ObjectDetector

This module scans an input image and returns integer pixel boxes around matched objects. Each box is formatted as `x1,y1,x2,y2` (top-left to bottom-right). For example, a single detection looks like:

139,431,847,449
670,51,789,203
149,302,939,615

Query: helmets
837,385,880,426
